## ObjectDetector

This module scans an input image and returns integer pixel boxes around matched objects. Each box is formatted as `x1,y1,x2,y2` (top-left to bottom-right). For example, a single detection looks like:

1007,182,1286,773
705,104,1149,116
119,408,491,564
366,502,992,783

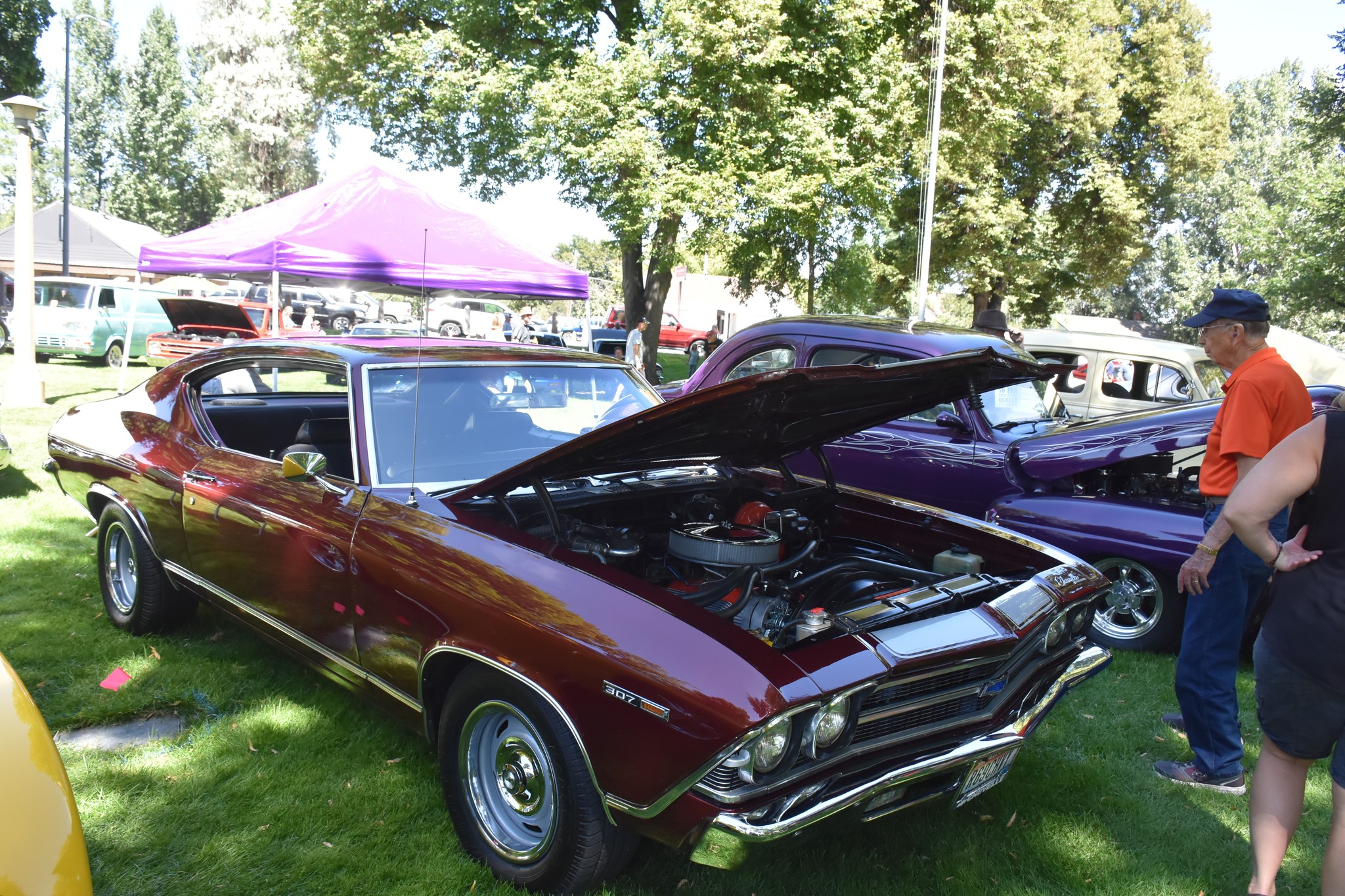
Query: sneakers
1152,759,1247,797
1161,712,1244,745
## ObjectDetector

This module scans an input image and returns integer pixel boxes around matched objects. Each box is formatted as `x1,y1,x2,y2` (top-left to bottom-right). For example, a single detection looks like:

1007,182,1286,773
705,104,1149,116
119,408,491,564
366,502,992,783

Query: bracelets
1263,541,1283,583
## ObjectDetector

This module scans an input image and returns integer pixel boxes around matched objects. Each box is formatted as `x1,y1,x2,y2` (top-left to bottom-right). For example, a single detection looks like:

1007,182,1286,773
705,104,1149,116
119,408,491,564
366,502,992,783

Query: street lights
3,92,50,357
63,12,112,268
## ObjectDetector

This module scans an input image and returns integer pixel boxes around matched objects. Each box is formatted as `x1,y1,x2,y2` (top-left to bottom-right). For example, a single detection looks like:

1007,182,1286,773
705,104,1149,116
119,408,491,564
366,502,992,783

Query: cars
994,329,1234,419
44,317,1113,894
494,316,663,382
311,286,424,338
145,295,324,372
0,271,43,353
583,321,1345,649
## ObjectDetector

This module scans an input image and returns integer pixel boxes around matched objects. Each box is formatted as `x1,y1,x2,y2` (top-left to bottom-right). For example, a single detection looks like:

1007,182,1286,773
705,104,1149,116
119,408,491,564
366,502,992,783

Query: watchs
1196,541,1221,556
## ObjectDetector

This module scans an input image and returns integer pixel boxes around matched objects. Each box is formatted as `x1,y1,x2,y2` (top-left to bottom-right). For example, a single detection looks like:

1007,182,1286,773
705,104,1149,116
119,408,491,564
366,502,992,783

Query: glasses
1198,323,1245,337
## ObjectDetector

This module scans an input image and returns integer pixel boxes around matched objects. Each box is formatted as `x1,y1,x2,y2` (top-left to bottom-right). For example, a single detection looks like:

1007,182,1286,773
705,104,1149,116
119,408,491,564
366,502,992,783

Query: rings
1192,580,1199,583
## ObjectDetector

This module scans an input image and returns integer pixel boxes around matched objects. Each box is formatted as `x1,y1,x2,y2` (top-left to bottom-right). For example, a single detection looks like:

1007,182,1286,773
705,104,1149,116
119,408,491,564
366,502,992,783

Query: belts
1205,496,1227,511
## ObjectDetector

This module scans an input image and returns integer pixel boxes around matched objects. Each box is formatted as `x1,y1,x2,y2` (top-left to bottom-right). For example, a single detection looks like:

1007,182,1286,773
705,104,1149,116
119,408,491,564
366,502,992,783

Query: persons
686,343,699,379
1117,360,1134,382
970,309,1025,354
613,346,625,362
301,307,315,328
612,321,624,330
1221,391,1345,896
281,306,297,328
485,312,507,342
623,317,651,372
703,331,723,361
510,306,535,345
312,320,326,336
1104,361,1114,375
1150,288,1315,798
503,312,513,331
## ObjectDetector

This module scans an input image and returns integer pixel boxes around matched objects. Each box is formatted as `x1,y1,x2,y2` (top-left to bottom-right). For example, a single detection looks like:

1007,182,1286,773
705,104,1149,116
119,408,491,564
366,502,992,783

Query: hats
312,320,320,327
614,346,623,351
636,316,650,325
504,313,513,318
1181,288,1271,328
972,309,1012,333
519,306,533,316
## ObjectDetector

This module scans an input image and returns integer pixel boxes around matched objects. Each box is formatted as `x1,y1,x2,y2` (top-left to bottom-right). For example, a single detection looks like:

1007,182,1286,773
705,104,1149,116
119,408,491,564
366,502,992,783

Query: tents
118,165,599,406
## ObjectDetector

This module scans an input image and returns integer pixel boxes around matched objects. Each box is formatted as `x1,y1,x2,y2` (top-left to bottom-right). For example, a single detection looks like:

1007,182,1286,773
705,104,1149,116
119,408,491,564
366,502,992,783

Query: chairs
274,416,353,478
453,411,534,453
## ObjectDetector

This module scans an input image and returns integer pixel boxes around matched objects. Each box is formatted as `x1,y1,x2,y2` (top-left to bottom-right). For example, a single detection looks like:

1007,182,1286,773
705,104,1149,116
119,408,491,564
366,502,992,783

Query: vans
33,276,182,369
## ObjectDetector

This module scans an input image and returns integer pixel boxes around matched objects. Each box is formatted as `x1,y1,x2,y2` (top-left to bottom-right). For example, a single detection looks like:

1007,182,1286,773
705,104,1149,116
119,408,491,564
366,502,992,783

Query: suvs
605,306,710,354
244,283,357,335
422,297,549,338
